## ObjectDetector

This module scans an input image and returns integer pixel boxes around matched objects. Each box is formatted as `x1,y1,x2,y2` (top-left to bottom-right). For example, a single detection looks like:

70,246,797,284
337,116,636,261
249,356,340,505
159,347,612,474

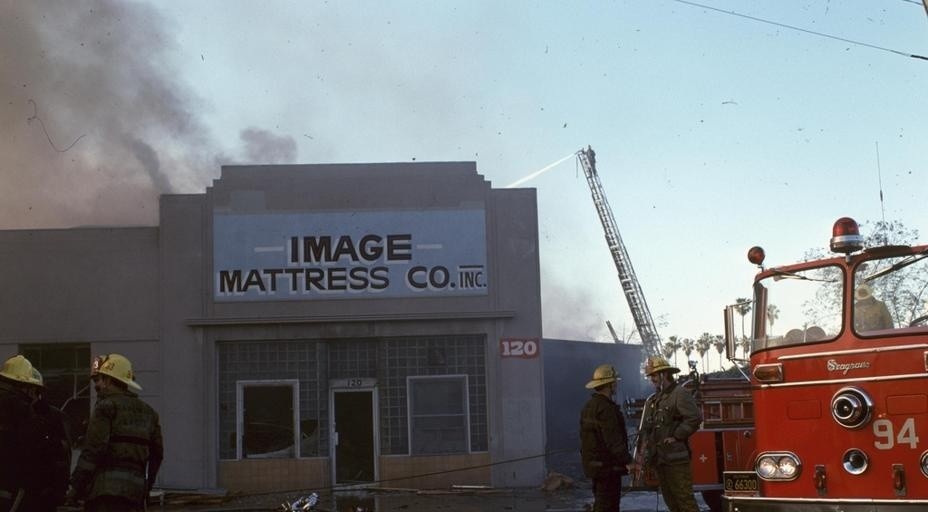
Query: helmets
89,351,144,392
585,363,623,391
643,356,681,378
0,354,45,387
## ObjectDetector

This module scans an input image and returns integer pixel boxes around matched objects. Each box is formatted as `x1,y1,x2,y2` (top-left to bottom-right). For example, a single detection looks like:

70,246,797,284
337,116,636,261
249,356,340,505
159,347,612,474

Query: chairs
783,324,826,344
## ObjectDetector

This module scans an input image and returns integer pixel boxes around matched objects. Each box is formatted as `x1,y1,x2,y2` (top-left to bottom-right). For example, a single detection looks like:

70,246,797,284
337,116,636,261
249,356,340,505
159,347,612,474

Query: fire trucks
574,149,928,512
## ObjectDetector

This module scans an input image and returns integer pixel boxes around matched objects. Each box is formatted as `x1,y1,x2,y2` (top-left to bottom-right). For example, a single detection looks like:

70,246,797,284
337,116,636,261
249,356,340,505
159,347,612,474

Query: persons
638,357,703,512
0,354,163,512
579,364,637,511
